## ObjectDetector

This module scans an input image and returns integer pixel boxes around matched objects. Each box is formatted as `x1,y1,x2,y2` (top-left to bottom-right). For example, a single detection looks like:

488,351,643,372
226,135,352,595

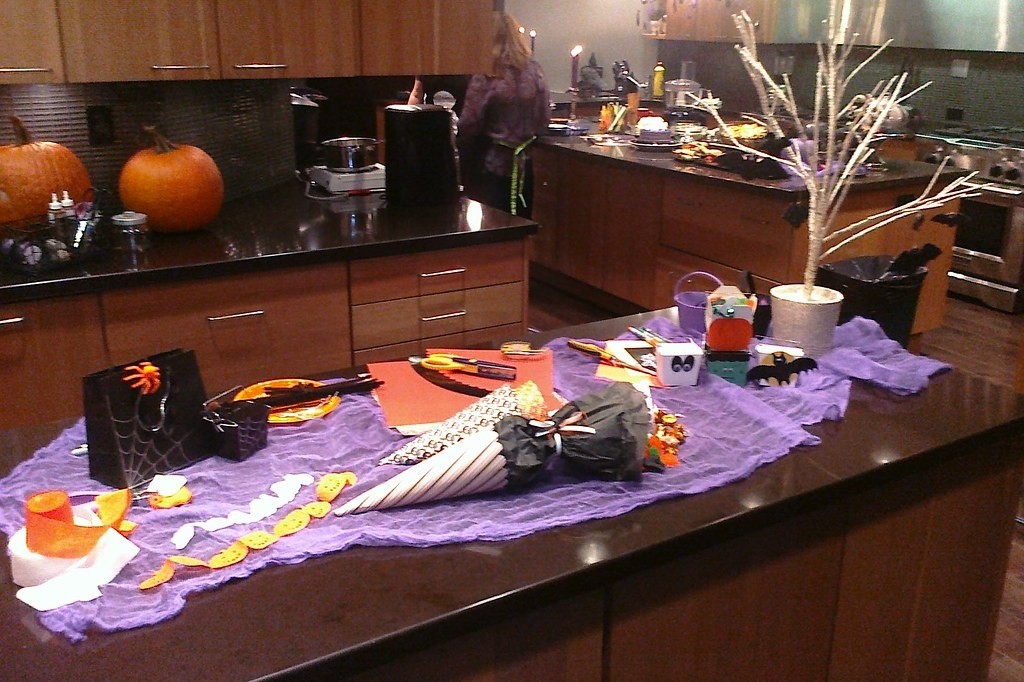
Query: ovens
923,139,1024,312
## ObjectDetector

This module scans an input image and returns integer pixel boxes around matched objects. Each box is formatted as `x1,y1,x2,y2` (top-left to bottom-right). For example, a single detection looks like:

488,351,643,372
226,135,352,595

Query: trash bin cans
817,254,928,348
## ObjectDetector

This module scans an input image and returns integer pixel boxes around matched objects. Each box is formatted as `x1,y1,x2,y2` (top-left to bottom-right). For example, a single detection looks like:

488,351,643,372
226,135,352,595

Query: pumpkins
120,126,224,232
0,116,94,228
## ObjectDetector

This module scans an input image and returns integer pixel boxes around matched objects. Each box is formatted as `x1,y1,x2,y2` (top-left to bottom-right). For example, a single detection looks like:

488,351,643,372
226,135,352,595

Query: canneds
111,211,150,253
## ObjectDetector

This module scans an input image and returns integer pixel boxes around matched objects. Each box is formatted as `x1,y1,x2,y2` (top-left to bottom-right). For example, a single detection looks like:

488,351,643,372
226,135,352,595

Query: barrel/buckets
673,272,724,339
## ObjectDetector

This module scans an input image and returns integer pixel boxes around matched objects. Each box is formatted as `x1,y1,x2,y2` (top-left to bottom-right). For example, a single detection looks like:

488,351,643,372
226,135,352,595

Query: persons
458,11,550,220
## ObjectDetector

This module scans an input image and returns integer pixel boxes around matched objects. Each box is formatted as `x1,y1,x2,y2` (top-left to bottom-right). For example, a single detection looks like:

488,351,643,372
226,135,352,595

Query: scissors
421,354,518,380
74,188,113,247
567,339,656,378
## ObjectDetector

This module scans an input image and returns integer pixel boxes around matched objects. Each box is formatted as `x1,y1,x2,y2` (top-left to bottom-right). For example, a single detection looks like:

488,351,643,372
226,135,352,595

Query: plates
630,139,684,151
233,378,340,424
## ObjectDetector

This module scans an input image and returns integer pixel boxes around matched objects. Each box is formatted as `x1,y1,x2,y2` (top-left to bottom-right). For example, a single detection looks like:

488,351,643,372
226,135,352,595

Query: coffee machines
384,104,464,212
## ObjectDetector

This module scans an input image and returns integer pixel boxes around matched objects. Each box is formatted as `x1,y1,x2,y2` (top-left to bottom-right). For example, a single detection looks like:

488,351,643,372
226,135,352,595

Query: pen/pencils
626,325,670,346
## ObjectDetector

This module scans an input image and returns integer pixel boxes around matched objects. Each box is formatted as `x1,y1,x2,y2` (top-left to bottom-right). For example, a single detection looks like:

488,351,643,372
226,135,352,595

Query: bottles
653,62,666,97
47,189,80,248
113,211,151,271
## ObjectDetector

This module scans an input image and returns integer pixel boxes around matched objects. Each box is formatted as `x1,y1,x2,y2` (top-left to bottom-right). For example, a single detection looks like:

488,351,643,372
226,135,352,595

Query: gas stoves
311,163,386,192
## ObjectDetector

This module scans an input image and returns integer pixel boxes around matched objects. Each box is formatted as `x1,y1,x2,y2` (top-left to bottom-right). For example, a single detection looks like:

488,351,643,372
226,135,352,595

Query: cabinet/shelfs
0,0,494,84
0,290,110,480
101,259,352,401
554,158,661,314
526,145,557,272
655,176,788,312
351,236,528,366
316,458,1024,682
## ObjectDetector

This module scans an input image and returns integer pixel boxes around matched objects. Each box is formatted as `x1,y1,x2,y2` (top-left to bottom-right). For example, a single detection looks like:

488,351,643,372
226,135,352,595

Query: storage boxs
705,347,748,388
755,343,804,388
702,285,759,334
203,398,273,462
654,342,703,386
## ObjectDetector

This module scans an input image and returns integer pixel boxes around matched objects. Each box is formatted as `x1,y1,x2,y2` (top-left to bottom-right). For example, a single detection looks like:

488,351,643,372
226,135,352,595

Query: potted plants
686,7,993,354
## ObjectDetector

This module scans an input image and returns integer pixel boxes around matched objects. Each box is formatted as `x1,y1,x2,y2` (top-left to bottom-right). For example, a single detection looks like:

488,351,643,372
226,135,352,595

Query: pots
321,137,383,168
